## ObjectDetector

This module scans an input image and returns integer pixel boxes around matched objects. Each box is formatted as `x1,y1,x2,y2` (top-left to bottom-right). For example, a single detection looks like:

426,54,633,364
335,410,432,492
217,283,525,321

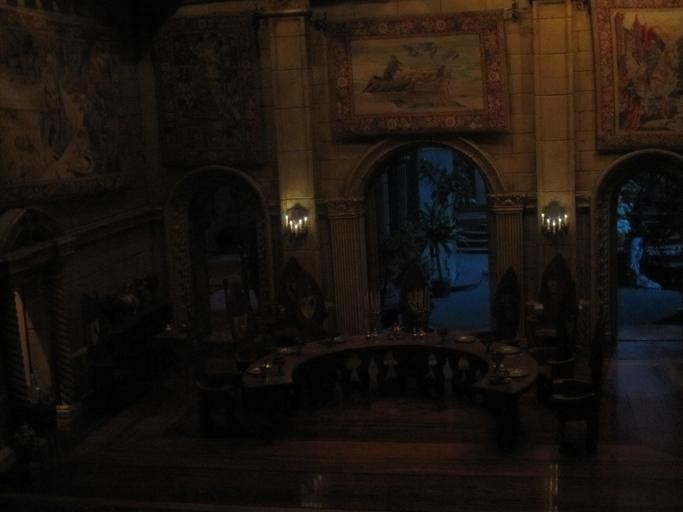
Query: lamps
541,201,570,249
284,203,310,247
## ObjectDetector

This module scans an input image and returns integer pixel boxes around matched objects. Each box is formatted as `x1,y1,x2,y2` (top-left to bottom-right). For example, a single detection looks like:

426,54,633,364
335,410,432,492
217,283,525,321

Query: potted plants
409,199,464,298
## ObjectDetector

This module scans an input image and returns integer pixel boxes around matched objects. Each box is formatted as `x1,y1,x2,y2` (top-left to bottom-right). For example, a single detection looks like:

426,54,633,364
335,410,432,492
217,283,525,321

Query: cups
249,357,287,385
489,353,511,385
411,327,425,340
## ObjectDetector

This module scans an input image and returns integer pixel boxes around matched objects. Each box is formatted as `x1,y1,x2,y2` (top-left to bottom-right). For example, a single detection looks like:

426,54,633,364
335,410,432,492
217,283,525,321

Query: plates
276,346,301,356
328,335,350,343
454,335,474,343
509,368,528,377
496,345,521,354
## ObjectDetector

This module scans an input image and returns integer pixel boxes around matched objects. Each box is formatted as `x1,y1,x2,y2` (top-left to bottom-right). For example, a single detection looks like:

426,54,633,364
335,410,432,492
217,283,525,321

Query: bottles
393,322,399,335
364,328,378,340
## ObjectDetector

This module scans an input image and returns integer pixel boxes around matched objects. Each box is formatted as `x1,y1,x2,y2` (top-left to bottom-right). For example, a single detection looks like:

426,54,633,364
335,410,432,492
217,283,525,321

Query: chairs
235,351,272,372
197,371,240,416
266,332,299,364
531,346,576,408
549,379,600,422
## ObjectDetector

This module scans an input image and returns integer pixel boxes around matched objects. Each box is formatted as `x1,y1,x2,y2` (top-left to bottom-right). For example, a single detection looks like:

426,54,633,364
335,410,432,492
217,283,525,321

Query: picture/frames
589,0,683,152
326,10,509,138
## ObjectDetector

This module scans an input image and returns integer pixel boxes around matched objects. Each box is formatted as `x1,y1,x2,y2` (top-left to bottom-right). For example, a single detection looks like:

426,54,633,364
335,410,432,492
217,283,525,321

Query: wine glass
481,336,494,354
436,328,449,345
